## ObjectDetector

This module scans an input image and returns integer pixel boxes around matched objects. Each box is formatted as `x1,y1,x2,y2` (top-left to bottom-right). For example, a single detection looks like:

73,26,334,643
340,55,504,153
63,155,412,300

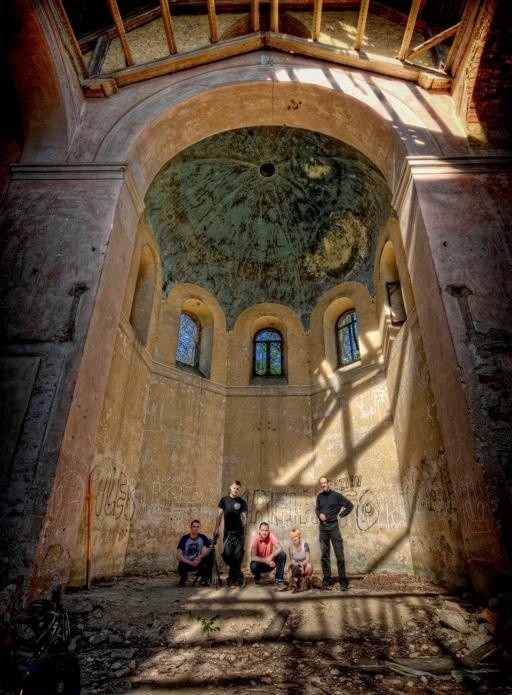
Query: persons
176,521,211,586
288,529,313,591
250,522,286,591
315,476,354,591
213,480,247,588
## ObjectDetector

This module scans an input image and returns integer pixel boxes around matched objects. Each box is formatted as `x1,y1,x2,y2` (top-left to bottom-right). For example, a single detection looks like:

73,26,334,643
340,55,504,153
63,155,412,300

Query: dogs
285,564,332,594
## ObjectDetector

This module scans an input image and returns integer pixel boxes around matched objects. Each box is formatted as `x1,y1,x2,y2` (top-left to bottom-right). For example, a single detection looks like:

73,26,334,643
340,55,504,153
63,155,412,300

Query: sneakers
178,576,188,586
227,574,246,588
254,578,261,585
340,585,348,591
275,578,284,585
199,580,210,587
